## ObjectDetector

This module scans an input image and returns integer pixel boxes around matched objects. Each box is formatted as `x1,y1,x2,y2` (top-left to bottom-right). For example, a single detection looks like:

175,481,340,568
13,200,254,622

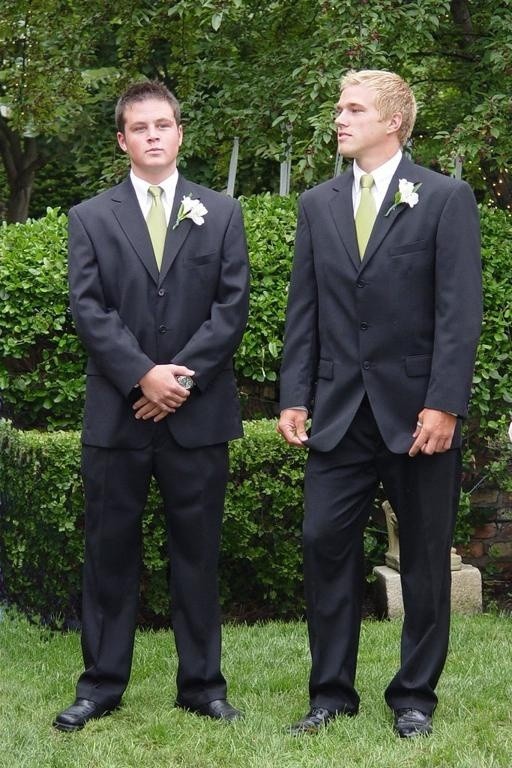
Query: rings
417,420,423,428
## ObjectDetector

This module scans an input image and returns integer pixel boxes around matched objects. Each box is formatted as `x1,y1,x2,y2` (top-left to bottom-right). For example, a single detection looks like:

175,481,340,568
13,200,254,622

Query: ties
354,173,378,261
144,186,167,273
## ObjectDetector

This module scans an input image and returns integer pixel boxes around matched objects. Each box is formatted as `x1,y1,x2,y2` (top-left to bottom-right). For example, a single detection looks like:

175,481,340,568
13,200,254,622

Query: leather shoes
395,706,434,741
284,707,340,737
172,696,245,723
52,696,111,734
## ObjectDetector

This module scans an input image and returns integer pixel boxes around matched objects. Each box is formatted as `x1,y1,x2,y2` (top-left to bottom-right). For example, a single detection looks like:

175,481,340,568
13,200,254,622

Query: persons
276,67,485,737
53,76,252,733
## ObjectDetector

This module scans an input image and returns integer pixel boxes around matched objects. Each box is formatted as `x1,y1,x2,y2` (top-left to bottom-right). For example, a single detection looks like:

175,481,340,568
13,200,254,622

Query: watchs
176,373,196,392
